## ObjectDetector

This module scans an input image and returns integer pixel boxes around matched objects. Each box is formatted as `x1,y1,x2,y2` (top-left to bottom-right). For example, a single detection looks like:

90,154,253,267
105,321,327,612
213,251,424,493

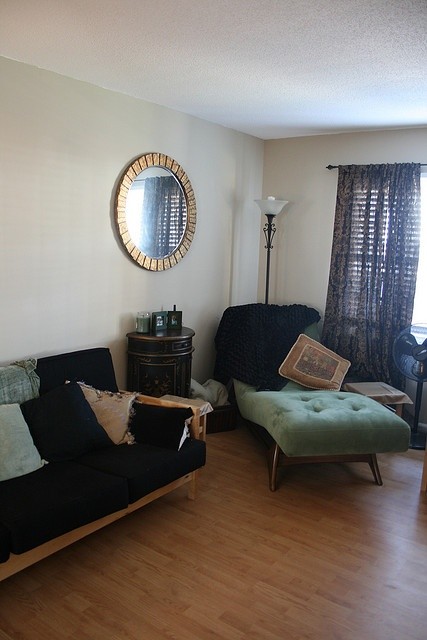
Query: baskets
206,399,239,436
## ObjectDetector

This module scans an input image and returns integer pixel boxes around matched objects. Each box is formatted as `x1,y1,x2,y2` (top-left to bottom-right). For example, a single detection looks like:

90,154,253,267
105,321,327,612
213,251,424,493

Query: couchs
0,347,214,584
214,303,411,491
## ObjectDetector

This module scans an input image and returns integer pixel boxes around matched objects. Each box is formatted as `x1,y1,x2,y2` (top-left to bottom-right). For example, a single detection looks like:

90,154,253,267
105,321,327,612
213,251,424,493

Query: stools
341,382,413,417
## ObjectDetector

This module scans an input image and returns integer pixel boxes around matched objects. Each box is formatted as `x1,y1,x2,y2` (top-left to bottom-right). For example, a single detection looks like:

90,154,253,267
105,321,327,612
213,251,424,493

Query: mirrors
115,151,200,272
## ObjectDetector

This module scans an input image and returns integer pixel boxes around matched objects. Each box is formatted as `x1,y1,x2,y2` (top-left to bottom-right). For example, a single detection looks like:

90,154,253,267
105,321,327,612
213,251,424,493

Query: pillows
20,381,117,458
65,381,138,445
0,404,49,481
278,333,351,392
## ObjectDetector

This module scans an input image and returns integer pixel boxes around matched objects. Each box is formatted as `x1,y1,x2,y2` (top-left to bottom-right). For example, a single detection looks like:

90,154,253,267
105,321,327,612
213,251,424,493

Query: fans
392,323,427,450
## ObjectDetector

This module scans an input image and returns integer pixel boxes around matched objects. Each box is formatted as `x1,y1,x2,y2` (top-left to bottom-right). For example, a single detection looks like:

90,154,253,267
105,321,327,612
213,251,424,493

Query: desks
125,327,196,399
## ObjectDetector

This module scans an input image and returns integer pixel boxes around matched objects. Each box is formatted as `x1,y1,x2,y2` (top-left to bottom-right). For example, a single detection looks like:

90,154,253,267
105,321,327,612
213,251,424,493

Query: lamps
254,196,289,304
